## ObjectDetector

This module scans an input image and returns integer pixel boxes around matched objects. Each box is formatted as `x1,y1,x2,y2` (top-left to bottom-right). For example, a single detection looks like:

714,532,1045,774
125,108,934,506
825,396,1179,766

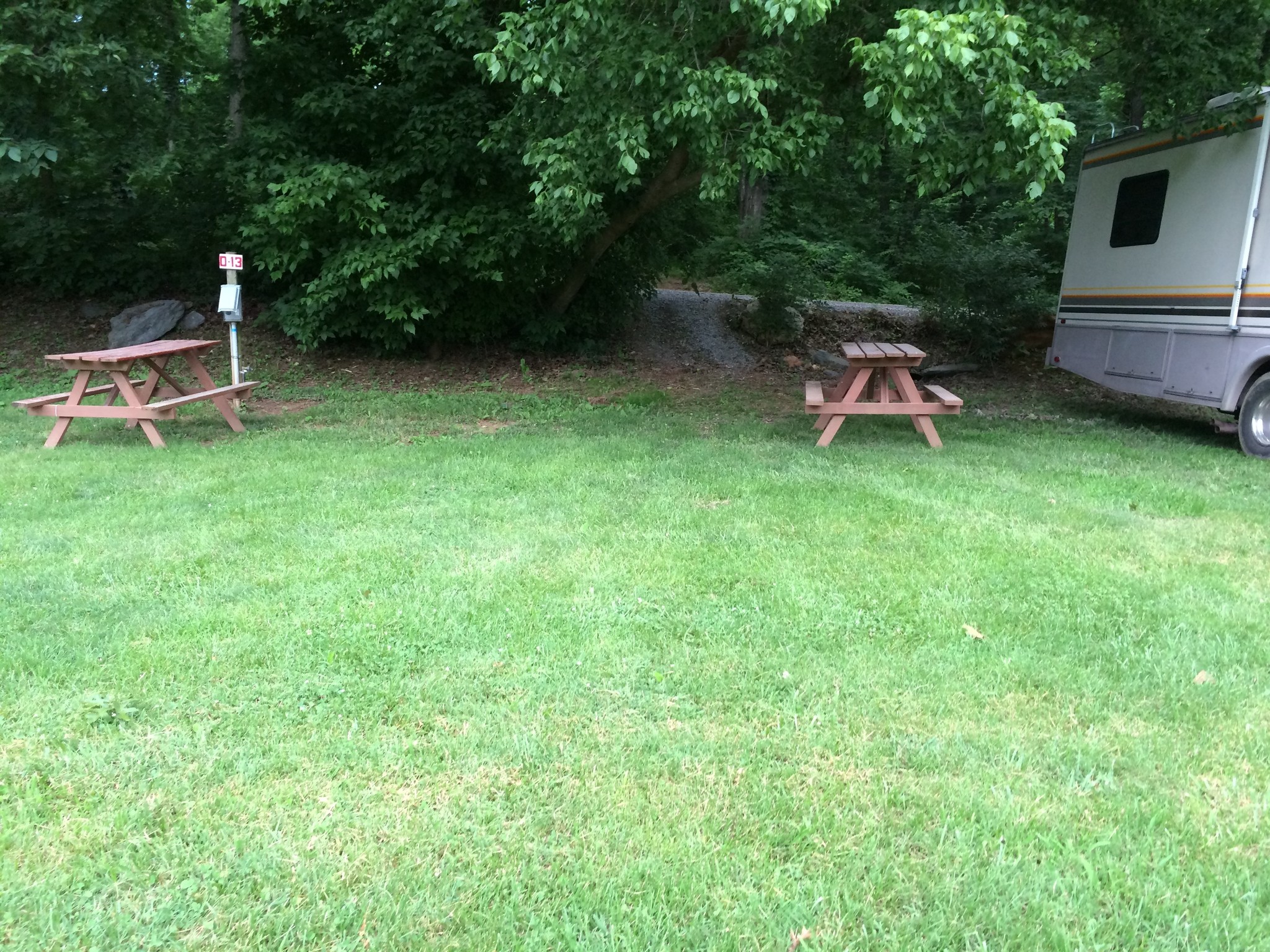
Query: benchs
12,380,146,409
923,385,963,415
141,382,261,412
805,381,824,414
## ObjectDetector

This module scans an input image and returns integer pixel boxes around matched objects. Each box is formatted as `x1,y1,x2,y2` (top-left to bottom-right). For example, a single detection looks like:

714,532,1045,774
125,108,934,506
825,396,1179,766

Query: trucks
1043,84,1270,459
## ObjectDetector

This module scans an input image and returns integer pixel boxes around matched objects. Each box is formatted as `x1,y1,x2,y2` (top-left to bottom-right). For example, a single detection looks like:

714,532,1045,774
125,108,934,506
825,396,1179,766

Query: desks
813,342,943,449
44,339,244,449
